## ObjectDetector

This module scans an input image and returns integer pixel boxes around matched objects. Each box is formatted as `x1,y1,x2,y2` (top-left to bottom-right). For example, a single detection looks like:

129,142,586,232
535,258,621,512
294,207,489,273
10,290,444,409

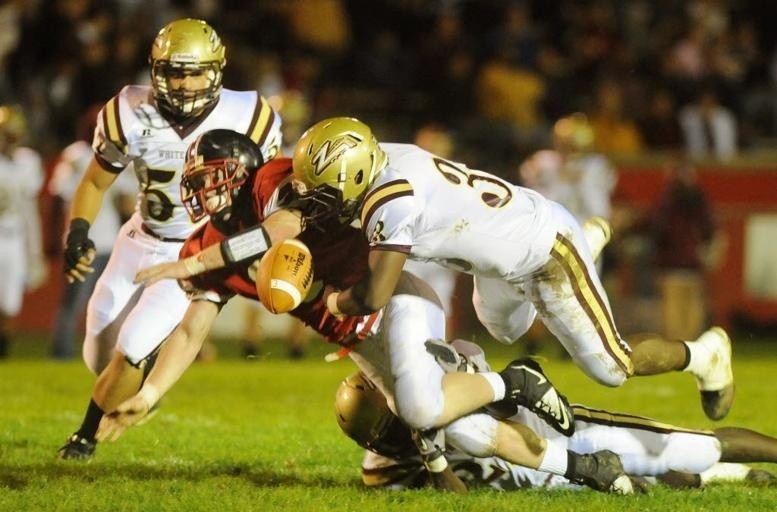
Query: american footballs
257,239,315,314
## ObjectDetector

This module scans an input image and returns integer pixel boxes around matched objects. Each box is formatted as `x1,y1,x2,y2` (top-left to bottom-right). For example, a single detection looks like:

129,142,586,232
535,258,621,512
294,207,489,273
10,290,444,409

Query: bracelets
184,255,205,275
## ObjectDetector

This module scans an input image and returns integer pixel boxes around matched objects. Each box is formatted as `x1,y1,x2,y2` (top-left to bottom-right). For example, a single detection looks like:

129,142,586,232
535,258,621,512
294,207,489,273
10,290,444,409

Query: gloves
63,217,95,273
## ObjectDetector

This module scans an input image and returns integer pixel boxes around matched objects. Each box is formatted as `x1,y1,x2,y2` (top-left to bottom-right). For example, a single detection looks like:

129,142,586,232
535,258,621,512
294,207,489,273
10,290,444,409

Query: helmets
333,371,424,462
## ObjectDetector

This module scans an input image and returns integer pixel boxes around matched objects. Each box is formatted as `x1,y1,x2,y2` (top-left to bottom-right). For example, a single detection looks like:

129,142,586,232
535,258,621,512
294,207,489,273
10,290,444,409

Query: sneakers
56,434,97,460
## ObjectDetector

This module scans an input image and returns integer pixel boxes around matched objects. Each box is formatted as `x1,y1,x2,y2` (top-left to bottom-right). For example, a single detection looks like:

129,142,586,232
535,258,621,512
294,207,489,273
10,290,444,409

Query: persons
297,112,738,420
95,126,578,444
56,17,285,463
1,2,775,357
327,293,349,323
334,365,776,498
